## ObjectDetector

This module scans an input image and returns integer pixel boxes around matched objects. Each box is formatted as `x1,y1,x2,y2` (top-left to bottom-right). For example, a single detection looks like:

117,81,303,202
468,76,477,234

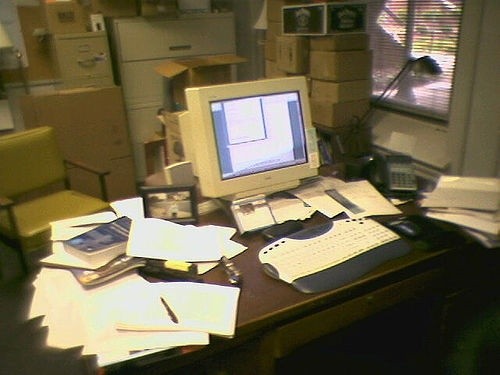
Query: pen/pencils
159,296,180,325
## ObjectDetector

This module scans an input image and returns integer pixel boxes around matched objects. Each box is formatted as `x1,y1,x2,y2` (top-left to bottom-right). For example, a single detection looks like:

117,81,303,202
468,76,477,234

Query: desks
88,191,499,375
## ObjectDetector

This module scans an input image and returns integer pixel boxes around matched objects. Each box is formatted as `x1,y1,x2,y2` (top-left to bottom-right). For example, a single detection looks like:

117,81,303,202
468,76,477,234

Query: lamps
342,55,444,148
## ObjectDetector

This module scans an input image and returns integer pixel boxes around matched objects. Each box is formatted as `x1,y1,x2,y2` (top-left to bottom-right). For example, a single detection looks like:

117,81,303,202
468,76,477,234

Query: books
68,252,147,286
39,251,124,269
61,215,136,263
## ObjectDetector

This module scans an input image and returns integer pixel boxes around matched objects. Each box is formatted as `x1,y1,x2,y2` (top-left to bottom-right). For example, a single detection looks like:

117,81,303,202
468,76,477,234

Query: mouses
385,218,421,237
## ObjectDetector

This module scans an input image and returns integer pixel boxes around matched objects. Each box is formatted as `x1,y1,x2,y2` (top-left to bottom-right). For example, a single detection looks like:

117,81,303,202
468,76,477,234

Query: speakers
163,160,198,191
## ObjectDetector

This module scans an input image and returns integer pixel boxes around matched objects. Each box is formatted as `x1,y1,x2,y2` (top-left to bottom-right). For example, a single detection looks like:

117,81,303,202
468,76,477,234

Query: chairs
0,126,110,277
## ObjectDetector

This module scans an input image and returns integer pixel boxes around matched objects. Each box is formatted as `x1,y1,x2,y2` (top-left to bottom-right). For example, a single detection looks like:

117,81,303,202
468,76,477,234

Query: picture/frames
139,183,200,226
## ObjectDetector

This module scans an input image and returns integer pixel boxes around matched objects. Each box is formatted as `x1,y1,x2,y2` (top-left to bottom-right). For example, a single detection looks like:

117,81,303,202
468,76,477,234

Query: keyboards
258,217,411,295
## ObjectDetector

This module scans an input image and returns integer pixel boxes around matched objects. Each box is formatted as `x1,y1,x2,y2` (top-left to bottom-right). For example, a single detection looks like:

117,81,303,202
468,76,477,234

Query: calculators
385,153,419,191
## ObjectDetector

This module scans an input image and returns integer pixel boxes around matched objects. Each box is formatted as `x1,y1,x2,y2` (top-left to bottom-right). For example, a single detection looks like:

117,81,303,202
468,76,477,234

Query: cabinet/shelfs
106,10,238,185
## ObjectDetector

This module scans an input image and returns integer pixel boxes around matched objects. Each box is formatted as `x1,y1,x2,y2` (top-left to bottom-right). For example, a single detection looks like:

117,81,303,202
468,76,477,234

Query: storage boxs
1,1,373,200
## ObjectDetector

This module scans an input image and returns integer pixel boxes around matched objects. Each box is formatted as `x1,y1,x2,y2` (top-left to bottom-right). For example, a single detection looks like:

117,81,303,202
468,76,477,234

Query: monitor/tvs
164,76,319,233
